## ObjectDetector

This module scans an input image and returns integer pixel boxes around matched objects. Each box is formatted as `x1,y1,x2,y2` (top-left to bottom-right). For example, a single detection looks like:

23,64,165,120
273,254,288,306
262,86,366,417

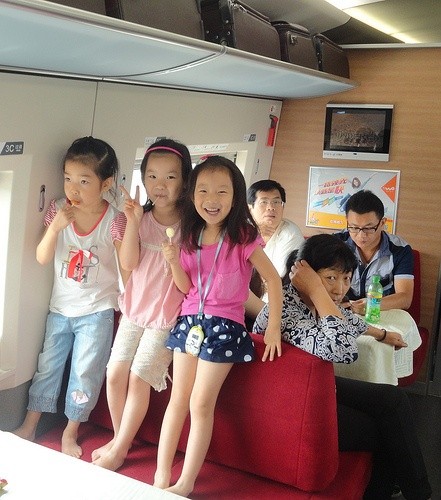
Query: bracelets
375,328,388,343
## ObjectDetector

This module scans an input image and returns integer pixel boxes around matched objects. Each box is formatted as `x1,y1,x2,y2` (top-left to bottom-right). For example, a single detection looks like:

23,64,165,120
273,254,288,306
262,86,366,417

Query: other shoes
378,486,402,497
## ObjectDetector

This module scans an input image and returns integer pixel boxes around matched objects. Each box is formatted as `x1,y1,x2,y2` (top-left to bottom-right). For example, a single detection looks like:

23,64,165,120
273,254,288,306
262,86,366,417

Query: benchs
32,244,430,500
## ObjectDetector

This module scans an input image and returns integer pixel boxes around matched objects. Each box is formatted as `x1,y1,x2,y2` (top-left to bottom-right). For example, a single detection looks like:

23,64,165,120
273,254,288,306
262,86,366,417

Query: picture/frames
306,166,401,236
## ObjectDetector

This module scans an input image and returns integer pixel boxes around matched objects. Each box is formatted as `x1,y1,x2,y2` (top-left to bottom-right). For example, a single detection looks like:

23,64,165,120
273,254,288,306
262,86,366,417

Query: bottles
365,274,382,323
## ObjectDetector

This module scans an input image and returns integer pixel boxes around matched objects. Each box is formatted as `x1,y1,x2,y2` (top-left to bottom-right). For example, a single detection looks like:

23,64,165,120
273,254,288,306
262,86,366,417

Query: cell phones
341,296,351,311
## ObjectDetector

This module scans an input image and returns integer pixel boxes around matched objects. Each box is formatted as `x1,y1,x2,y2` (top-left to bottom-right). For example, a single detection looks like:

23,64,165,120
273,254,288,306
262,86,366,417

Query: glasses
346,220,381,234
252,199,285,207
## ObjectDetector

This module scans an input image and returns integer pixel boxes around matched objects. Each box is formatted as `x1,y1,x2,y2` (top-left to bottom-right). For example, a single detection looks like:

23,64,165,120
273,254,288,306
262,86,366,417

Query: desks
0,429,193,500
261,289,423,387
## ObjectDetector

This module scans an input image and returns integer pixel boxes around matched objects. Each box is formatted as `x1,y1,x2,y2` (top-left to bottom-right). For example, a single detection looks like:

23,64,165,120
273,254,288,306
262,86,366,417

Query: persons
329,189,415,317
149,153,282,498
250,233,434,500
244,179,305,333
91,139,194,472
8,135,134,459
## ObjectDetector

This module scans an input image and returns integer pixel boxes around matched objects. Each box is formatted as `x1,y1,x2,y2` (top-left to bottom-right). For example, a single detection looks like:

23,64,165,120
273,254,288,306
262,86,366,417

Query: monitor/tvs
322,103,394,161
